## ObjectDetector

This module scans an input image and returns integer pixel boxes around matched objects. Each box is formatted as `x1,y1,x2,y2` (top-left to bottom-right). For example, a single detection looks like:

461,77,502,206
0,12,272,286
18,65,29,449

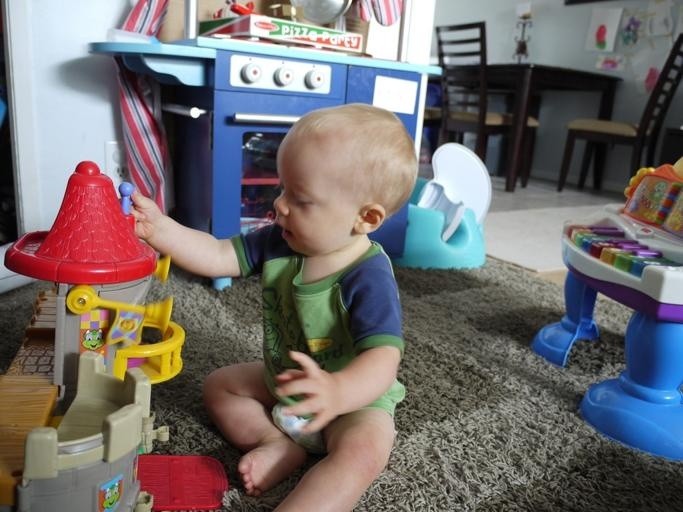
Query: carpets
1,252,682,512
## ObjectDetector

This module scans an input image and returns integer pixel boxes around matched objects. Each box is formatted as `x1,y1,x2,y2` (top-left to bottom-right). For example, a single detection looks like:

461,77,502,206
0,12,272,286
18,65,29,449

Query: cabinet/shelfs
173,49,426,291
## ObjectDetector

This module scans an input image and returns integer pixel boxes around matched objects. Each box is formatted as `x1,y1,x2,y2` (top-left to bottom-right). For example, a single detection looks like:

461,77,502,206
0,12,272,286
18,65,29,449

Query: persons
126,101,419,512
